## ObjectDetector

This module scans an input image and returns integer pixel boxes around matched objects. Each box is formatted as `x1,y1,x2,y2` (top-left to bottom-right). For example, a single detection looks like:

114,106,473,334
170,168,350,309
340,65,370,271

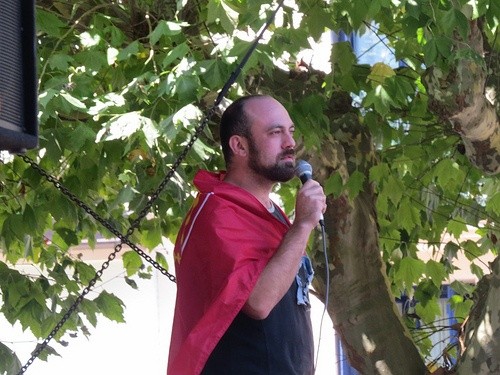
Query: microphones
295,159,325,227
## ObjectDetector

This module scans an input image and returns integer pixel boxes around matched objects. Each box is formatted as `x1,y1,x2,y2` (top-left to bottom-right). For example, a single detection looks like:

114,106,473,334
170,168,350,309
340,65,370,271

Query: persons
159,92,331,374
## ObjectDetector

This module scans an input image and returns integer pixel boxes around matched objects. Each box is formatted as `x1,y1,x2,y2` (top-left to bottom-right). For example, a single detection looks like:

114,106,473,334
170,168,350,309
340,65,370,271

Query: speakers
0,0,40,152
